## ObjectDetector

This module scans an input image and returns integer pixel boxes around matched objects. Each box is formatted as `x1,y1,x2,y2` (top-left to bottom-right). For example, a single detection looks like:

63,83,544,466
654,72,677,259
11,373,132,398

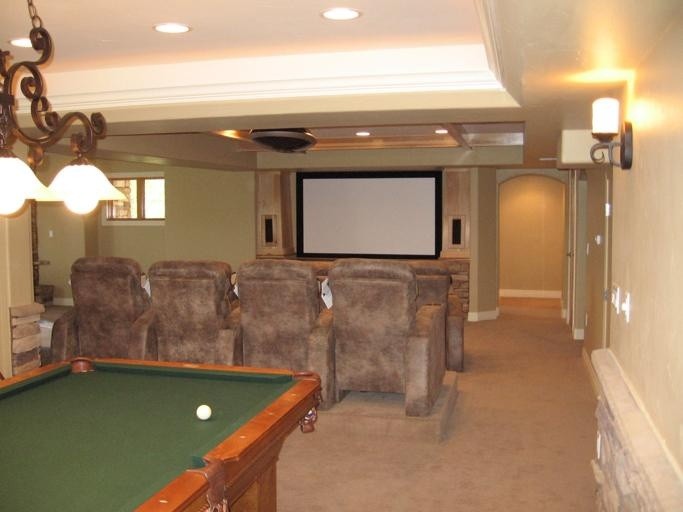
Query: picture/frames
0,352,322,512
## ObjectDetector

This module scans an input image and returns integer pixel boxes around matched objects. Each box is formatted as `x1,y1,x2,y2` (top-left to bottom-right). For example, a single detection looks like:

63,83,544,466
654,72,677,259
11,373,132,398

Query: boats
452,219,461,245
265,219,273,242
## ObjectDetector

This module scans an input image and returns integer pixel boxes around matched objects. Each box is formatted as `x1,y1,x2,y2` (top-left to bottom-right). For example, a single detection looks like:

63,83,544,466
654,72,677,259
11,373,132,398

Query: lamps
588,96,633,169
0,0,128,217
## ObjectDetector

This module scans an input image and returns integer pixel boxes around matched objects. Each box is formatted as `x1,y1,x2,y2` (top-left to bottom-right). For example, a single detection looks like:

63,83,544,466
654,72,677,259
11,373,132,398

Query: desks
0,352,322,512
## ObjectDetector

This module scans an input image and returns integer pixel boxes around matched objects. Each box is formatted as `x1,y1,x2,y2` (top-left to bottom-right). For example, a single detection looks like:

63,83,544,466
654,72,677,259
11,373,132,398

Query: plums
248,128,318,154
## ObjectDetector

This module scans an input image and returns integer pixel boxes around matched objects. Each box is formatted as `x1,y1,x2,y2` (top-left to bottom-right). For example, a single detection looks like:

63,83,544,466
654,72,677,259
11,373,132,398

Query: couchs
49,256,464,417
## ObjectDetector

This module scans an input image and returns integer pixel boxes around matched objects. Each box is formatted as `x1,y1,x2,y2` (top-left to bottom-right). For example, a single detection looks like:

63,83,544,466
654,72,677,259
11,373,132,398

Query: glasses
0,0,128,217
588,96,633,169
